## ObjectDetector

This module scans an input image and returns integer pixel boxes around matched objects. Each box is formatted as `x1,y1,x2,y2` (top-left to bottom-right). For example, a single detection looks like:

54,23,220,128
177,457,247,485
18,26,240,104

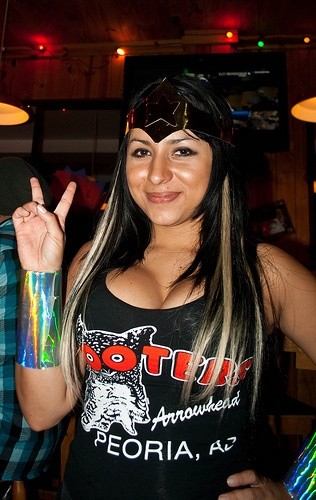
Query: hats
0,157,52,216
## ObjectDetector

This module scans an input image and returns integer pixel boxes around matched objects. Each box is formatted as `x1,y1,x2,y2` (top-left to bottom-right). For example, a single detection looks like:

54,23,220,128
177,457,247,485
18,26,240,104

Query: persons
0,156,71,500
12,77,316,500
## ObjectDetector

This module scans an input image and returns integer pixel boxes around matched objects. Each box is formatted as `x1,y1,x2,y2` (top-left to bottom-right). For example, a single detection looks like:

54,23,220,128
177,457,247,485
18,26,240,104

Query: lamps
0,0,32,127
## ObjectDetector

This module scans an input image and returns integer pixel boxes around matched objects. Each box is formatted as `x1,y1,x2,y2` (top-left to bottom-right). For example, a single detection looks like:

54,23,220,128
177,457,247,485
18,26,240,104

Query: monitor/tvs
119,51,290,153
